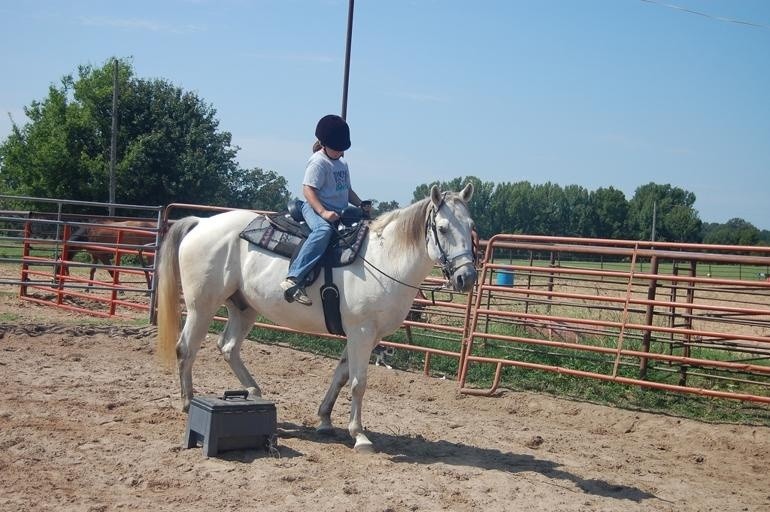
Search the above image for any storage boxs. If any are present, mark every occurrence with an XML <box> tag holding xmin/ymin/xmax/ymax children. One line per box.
<box><xmin>182</xmin><ymin>390</ymin><xmax>277</xmax><ymax>455</ymax></box>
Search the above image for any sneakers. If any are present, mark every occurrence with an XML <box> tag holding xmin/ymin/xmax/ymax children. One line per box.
<box><xmin>279</xmin><ymin>278</ymin><xmax>312</xmax><ymax>305</ymax></box>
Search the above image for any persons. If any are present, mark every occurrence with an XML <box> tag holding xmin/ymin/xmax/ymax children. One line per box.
<box><xmin>280</xmin><ymin>115</ymin><xmax>361</xmax><ymax>306</ymax></box>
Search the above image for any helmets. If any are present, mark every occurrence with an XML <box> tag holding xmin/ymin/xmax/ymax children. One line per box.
<box><xmin>315</xmin><ymin>115</ymin><xmax>350</xmax><ymax>151</ymax></box>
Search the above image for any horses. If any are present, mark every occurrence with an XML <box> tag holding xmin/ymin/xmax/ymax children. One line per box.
<box><xmin>156</xmin><ymin>182</ymin><xmax>478</xmax><ymax>455</ymax></box>
<box><xmin>50</xmin><ymin>217</ymin><xmax>170</xmax><ymax>296</ymax></box>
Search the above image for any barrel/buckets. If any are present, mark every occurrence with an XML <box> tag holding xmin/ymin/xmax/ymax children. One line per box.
<box><xmin>498</xmin><ymin>269</ymin><xmax>514</xmax><ymax>287</ymax></box>
